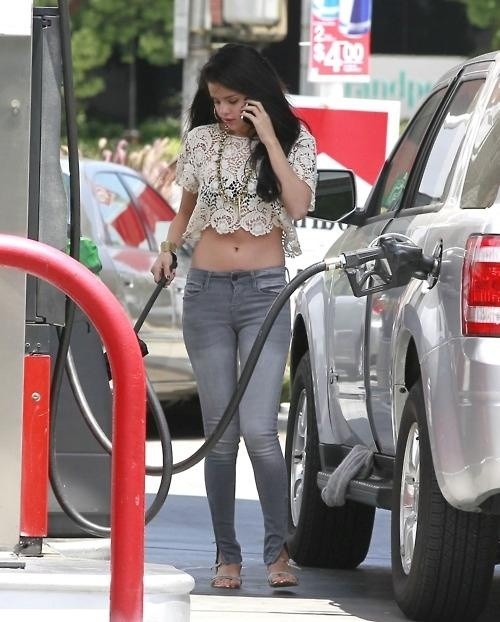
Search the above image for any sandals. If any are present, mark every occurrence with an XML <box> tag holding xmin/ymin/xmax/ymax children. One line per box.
<box><xmin>210</xmin><ymin>563</ymin><xmax>242</xmax><ymax>590</ymax></box>
<box><xmin>266</xmin><ymin>557</ymin><xmax>298</xmax><ymax>588</ymax></box>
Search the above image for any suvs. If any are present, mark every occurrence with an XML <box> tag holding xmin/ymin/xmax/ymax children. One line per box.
<box><xmin>279</xmin><ymin>50</ymin><xmax>500</xmax><ymax>622</ymax></box>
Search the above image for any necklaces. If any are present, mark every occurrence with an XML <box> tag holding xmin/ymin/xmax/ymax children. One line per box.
<box><xmin>217</xmin><ymin>123</ymin><xmax>255</xmax><ymax>201</ymax></box>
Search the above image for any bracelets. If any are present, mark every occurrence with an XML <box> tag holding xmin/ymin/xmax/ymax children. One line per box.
<box><xmin>160</xmin><ymin>242</ymin><xmax>178</xmax><ymax>255</ymax></box>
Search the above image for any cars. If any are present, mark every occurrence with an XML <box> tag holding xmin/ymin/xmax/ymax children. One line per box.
<box><xmin>59</xmin><ymin>156</ymin><xmax>197</xmax><ymax>406</ymax></box>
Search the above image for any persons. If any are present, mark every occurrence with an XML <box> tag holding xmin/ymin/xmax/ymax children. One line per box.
<box><xmin>151</xmin><ymin>42</ymin><xmax>318</xmax><ymax>589</ymax></box>
